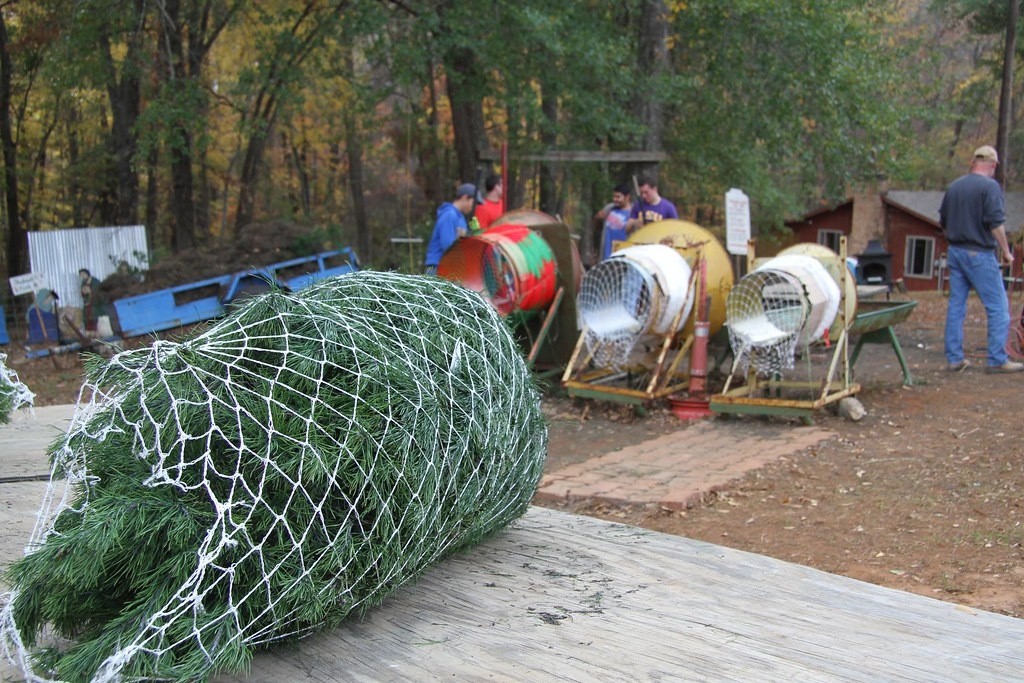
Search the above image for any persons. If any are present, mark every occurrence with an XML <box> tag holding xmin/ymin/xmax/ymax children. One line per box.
<box><xmin>465</xmin><ymin>172</ymin><xmax>502</xmax><ymax>234</ymax></box>
<box><xmin>424</xmin><ymin>182</ymin><xmax>484</xmax><ymax>277</ymax></box>
<box><xmin>593</xmin><ymin>183</ymin><xmax>643</xmax><ymax>263</ymax></box>
<box><xmin>937</xmin><ymin>143</ymin><xmax>1023</xmax><ymax>374</ymax></box>
<box><xmin>624</xmin><ymin>174</ymin><xmax>677</xmax><ymax>236</ymax></box>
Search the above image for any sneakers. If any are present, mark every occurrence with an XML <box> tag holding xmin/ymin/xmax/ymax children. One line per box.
<box><xmin>987</xmin><ymin>360</ymin><xmax>1023</xmax><ymax>373</ymax></box>
<box><xmin>949</xmin><ymin>359</ymin><xmax>969</xmax><ymax>372</ymax></box>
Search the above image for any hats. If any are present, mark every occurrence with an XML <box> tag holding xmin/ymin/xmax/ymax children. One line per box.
<box><xmin>456</xmin><ymin>183</ymin><xmax>483</xmax><ymax>204</ymax></box>
<box><xmin>974</xmin><ymin>145</ymin><xmax>1000</xmax><ymax>166</ymax></box>
